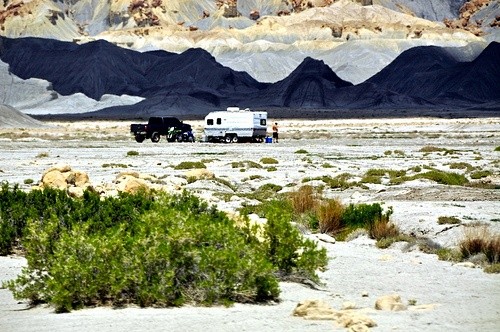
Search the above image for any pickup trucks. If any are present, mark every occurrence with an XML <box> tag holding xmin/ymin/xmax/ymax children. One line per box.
<box><xmin>130</xmin><ymin>116</ymin><xmax>191</xmax><ymax>142</ymax></box>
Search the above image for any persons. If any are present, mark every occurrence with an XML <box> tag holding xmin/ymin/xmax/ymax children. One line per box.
<box><xmin>272</xmin><ymin>122</ymin><xmax>279</xmax><ymax>143</ymax></box>
